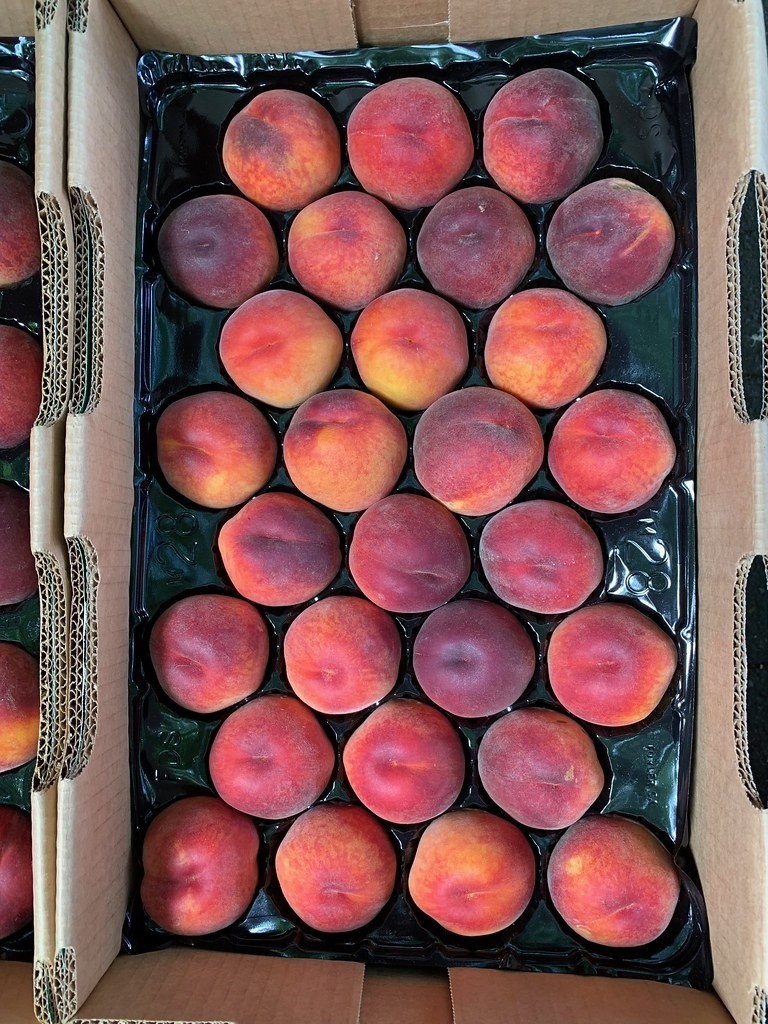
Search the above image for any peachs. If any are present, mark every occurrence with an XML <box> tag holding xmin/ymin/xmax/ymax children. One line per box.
<box><xmin>140</xmin><ymin>66</ymin><xmax>679</xmax><ymax>948</ymax></box>
<box><xmin>0</xmin><ymin>158</ymin><xmax>36</xmax><ymax>940</ymax></box>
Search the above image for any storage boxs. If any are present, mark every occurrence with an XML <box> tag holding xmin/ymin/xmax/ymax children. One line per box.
<box><xmin>0</xmin><ymin>0</ymin><xmax>768</xmax><ymax>1024</ymax></box>
